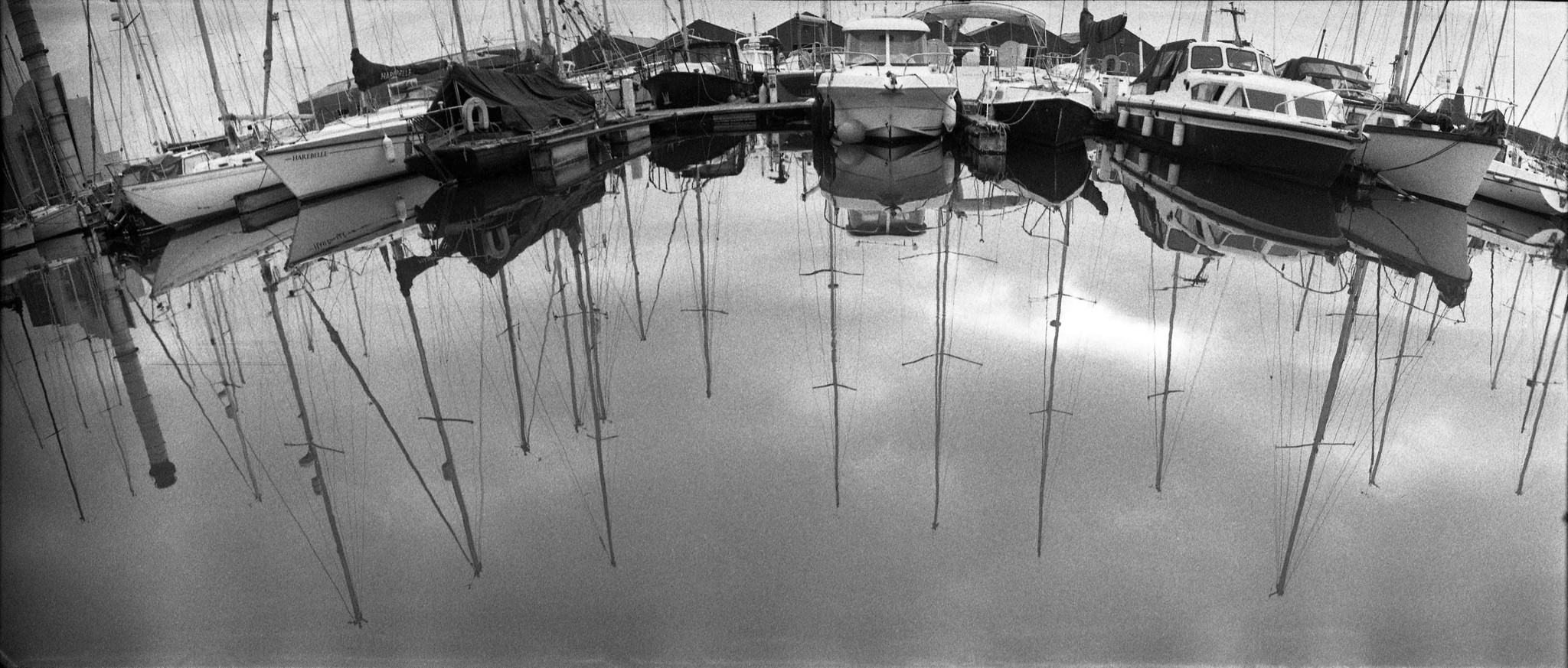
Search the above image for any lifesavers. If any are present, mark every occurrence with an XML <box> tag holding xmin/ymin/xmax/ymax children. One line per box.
<box><xmin>462</xmin><ymin>96</ymin><xmax>488</xmax><ymax>132</ymax></box>
<box><xmin>1101</xmin><ymin>55</ymin><xmax>1121</xmax><ymax>74</ymax></box>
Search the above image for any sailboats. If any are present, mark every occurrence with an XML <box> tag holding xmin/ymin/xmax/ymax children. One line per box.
<box><xmin>2</xmin><ymin>2</ymin><xmax>1568</xmax><ymax>250</ymax></box>
<box><xmin>2</xmin><ymin>139</ymin><xmax>1564</xmax><ymax>630</ymax></box>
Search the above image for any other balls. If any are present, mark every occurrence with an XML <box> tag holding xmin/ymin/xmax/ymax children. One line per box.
<box><xmin>837</xmin><ymin>118</ymin><xmax>864</xmax><ymax>142</ymax></box>
